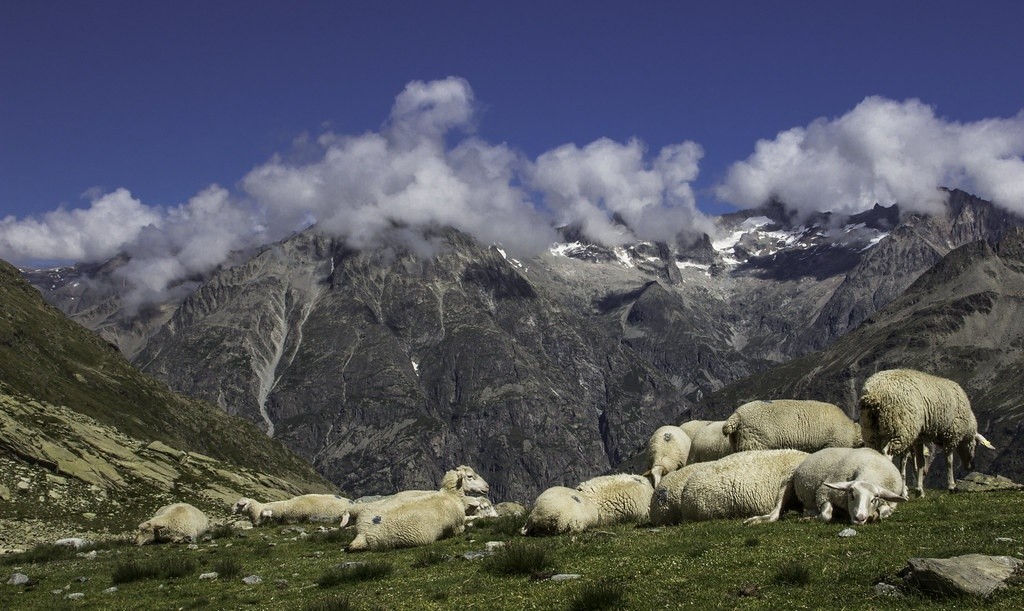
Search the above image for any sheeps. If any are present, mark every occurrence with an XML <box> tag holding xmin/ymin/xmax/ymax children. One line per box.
<box><xmin>134</xmin><ymin>502</ymin><xmax>209</xmax><ymax>546</ymax></box>
<box><xmin>230</xmin><ymin>368</ymin><xmax>997</xmax><ymax>552</ymax></box>
